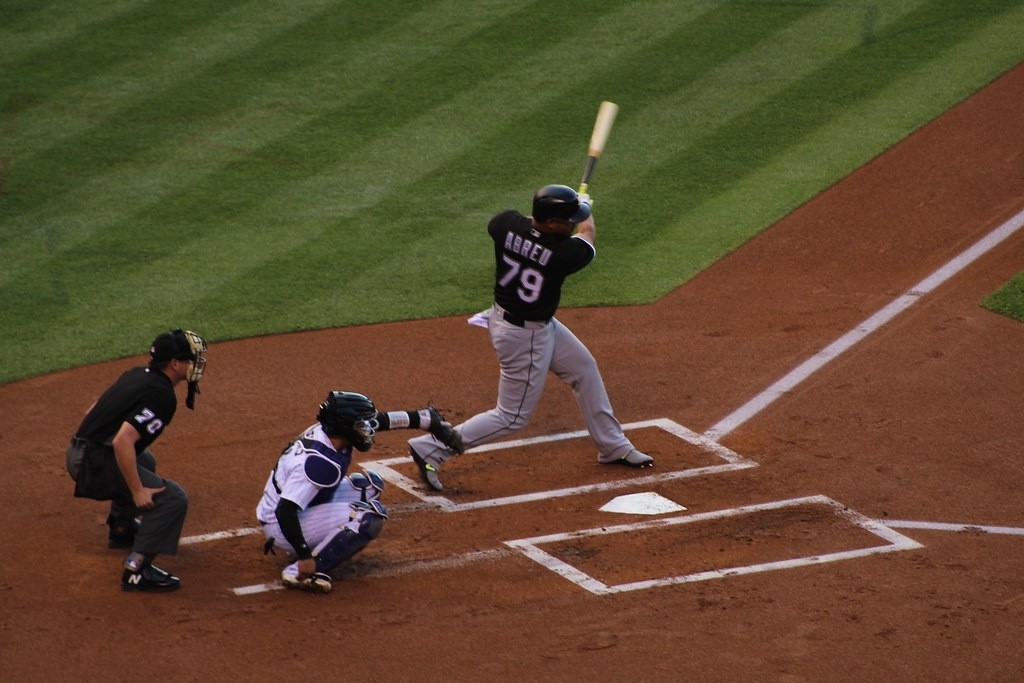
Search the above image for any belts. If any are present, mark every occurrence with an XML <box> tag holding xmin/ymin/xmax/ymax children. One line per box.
<box><xmin>260</xmin><ymin>521</ymin><xmax>267</xmax><ymax>526</ymax></box>
<box><xmin>72</xmin><ymin>437</ymin><xmax>87</xmax><ymax>449</ymax></box>
<box><xmin>493</xmin><ymin>301</ymin><xmax>553</xmax><ymax>327</ymax></box>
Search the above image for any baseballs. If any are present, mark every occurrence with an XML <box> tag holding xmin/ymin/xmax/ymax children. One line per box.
<box><xmin>579</xmin><ymin>98</ymin><xmax>622</xmax><ymax>193</ymax></box>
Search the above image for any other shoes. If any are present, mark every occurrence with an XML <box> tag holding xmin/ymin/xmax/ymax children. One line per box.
<box><xmin>282</xmin><ymin>564</ymin><xmax>333</xmax><ymax>594</ymax></box>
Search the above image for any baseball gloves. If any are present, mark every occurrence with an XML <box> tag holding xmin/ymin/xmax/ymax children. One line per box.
<box><xmin>430</xmin><ymin>409</ymin><xmax>466</xmax><ymax>455</ymax></box>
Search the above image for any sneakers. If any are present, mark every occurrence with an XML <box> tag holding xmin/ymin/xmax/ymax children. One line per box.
<box><xmin>121</xmin><ymin>562</ymin><xmax>181</xmax><ymax>592</ymax></box>
<box><xmin>619</xmin><ymin>447</ymin><xmax>655</xmax><ymax>468</ymax></box>
<box><xmin>109</xmin><ymin>518</ymin><xmax>143</xmax><ymax>548</ymax></box>
<box><xmin>407</xmin><ymin>443</ymin><xmax>443</xmax><ymax>492</ymax></box>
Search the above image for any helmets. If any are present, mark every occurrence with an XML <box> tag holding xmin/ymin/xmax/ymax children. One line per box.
<box><xmin>532</xmin><ymin>184</ymin><xmax>591</xmax><ymax>224</ymax></box>
<box><xmin>315</xmin><ymin>388</ymin><xmax>380</xmax><ymax>452</ymax></box>
<box><xmin>149</xmin><ymin>328</ymin><xmax>195</xmax><ymax>370</ymax></box>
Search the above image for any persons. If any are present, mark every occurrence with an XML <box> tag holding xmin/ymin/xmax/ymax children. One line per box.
<box><xmin>256</xmin><ymin>390</ymin><xmax>465</xmax><ymax>592</ymax></box>
<box><xmin>407</xmin><ymin>184</ymin><xmax>654</xmax><ymax>489</ymax></box>
<box><xmin>66</xmin><ymin>329</ymin><xmax>207</xmax><ymax>591</ymax></box>
